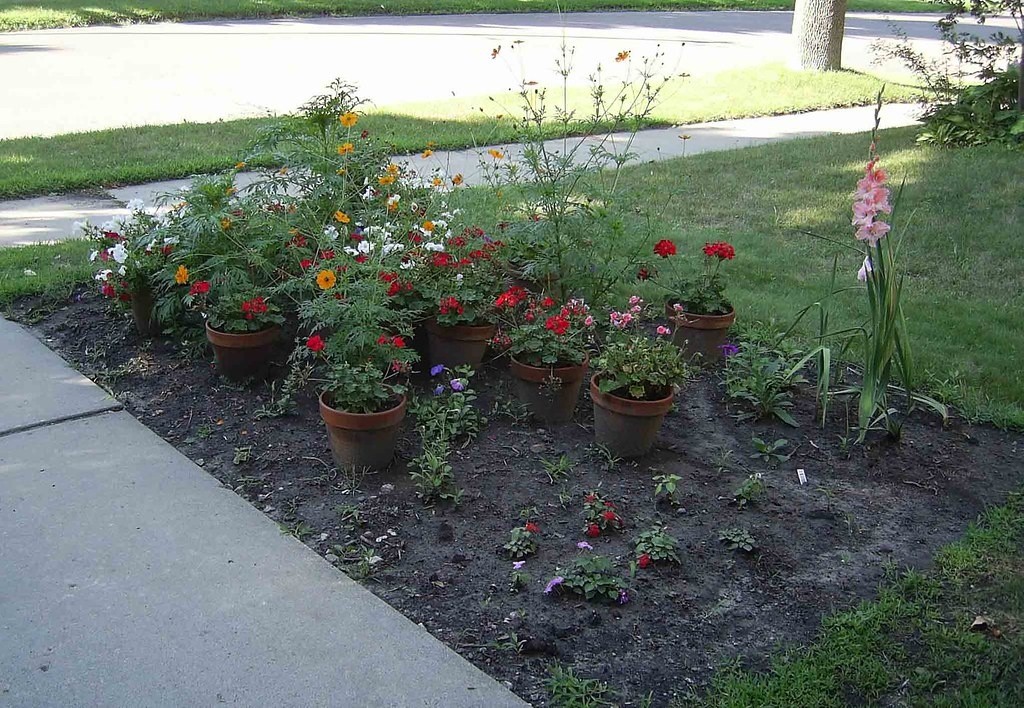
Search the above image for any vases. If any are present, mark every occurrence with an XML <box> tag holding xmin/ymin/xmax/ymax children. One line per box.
<box><xmin>589</xmin><ymin>373</ymin><xmax>674</xmax><ymax>458</ymax></box>
<box><xmin>130</xmin><ymin>291</ymin><xmax>180</xmax><ymax>353</ymax></box>
<box><xmin>664</xmin><ymin>295</ymin><xmax>734</xmax><ymax>365</ymax></box>
<box><xmin>508</xmin><ymin>348</ymin><xmax>586</xmax><ymax>422</ymax></box>
<box><xmin>205</xmin><ymin>315</ymin><xmax>276</xmax><ymax>375</ymax></box>
<box><xmin>503</xmin><ymin>260</ymin><xmax>558</xmax><ymax>292</ymax></box>
<box><xmin>318</xmin><ymin>381</ymin><xmax>407</xmax><ymax>473</ymax></box>
<box><xmin>419</xmin><ymin>315</ymin><xmax>493</xmax><ymax>375</ymax></box>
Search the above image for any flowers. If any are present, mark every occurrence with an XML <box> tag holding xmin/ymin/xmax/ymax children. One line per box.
<box><xmin>177</xmin><ymin>250</ymin><xmax>273</xmax><ymax>314</ymax></box>
<box><xmin>496</xmin><ymin>201</ymin><xmax>548</xmax><ymax>257</ymax></box>
<box><xmin>411</xmin><ymin>205</ymin><xmax>502</xmax><ymax>314</ymax></box>
<box><xmin>582</xmin><ymin>295</ymin><xmax>705</xmax><ymax>399</ymax></box>
<box><xmin>489</xmin><ymin>286</ymin><xmax>581</xmax><ymax>347</ymax></box>
<box><xmin>301</xmin><ymin>268</ymin><xmax>418</xmax><ymax>381</ymax></box>
<box><xmin>637</xmin><ymin>239</ymin><xmax>736</xmax><ymax>294</ymax></box>
<box><xmin>95</xmin><ymin>201</ymin><xmax>161</xmax><ymax>300</ymax></box>
